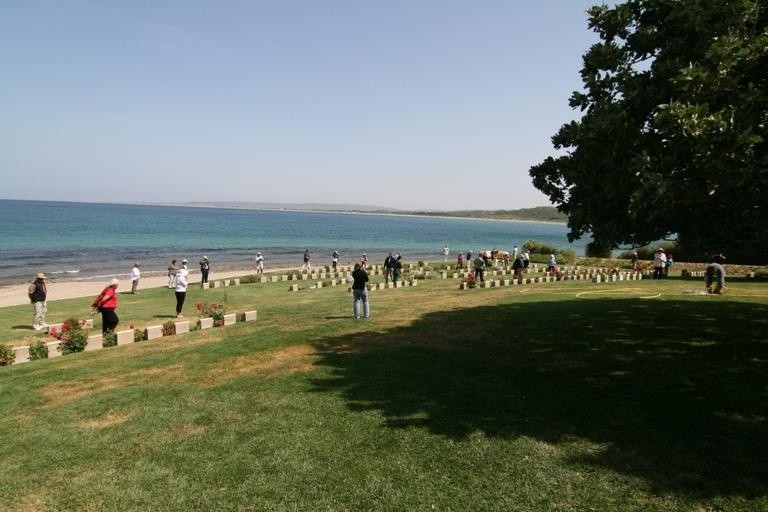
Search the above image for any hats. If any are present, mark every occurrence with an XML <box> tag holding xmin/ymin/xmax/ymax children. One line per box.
<box><xmin>177</xmin><ymin>269</ymin><xmax>187</xmax><ymax>275</ymax></box>
<box><xmin>107</xmin><ymin>278</ymin><xmax>119</xmax><ymax>285</ymax></box>
<box><xmin>34</xmin><ymin>274</ymin><xmax>47</xmax><ymax>278</ymax></box>
<box><xmin>656</xmin><ymin>248</ymin><xmax>664</xmax><ymax>251</ymax></box>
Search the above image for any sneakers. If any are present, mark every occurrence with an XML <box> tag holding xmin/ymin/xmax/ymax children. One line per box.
<box><xmin>33</xmin><ymin>324</ymin><xmax>47</xmax><ymax>330</ymax></box>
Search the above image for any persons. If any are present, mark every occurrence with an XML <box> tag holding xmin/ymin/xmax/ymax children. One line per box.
<box><xmin>653</xmin><ymin>248</ymin><xmax>667</xmax><ymax>279</ymax></box>
<box><xmin>131</xmin><ymin>262</ymin><xmax>142</xmax><ymax>294</ymax></box>
<box><xmin>168</xmin><ymin>258</ymin><xmax>190</xmax><ymax>319</ymax></box>
<box><xmin>632</xmin><ymin>252</ymin><xmax>638</xmax><ymax>271</ymax></box>
<box><xmin>712</xmin><ymin>250</ymin><xmax>727</xmax><ymax>265</ymax></box>
<box><xmin>255</xmin><ymin>252</ymin><xmax>264</xmax><ymax>277</ymax></box>
<box><xmin>28</xmin><ymin>273</ymin><xmax>49</xmax><ymax>330</ymax></box>
<box><xmin>706</xmin><ymin>262</ymin><xmax>726</xmax><ymax>294</ymax></box>
<box><xmin>455</xmin><ymin>245</ymin><xmax>529</xmax><ymax>283</ymax></box>
<box><xmin>302</xmin><ymin>248</ymin><xmax>312</xmax><ymax>271</ymax></box>
<box><xmin>548</xmin><ymin>254</ymin><xmax>556</xmax><ymax>271</ymax></box>
<box><xmin>352</xmin><ymin>252</ymin><xmax>370</xmax><ymax>319</ymax></box>
<box><xmin>332</xmin><ymin>250</ymin><xmax>339</xmax><ymax>268</ymax></box>
<box><xmin>382</xmin><ymin>251</ymin><xmax>404</xmax><ymax>288</ymax></box>
<box><xmin>199</xmin><ymin>256</ymin><xmax>210</xmax><ymax>283</ymax></box>
<box><xmin>441</xmin><ymin>244</ymin><xmax>449</xmax><ymax>263</ymax></box>
<box><xmin>91</xmin><ymin>278</ymin><xmax>119</xmax><ymax>338</ymax></box>
<box><xmin>665</xmin><ymin>253</ymin><xmax>673</xmax><ymax>278</ymax></box>
